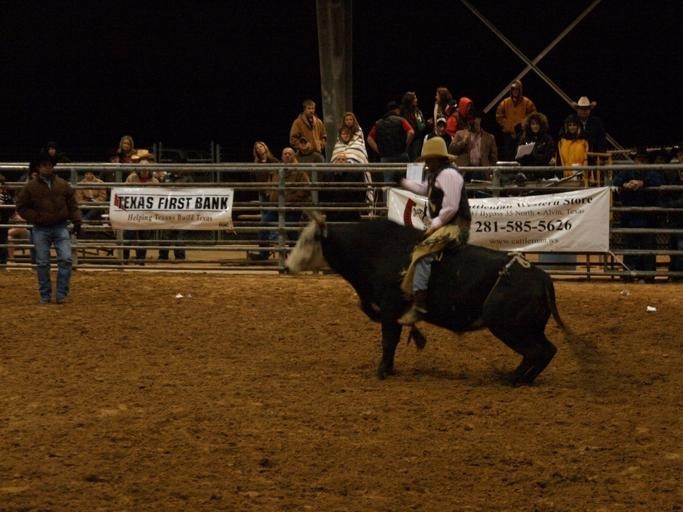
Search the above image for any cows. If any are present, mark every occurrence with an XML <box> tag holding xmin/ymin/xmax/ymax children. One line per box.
<box><xmin>282</xmin><ymin>206</ymin><xmax>565</xmax><ymax>388</ymax></box>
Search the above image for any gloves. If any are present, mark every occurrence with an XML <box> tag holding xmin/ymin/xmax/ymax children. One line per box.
<box><xmin>72</xmin><ymin>224</ymin><xmax>80</xmax><ymax>237</ymax></box>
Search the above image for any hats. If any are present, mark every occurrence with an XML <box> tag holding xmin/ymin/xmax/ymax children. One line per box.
<box><xmin>30</xmin><ymin>153</ymin><xmax>57</xmax><ymax>169</ymax></box>
<box><xmin>630</xmin><ymin>148</ymin><xmax>683</xmax><ymax>158</ymax></box>
<box><xmin>416</xmin><ymin>137</ymin><xmax>453</xmax><ymax>159</ymax></box>
<box><xmin>132</xmin><ymin>149</ymin><xmax>151</xmax><ymax>159</ymax></box>
<box><xmin>571</xmin><ymin>96</ymin><xmax>596</xmax><ymax>110</ymax></box>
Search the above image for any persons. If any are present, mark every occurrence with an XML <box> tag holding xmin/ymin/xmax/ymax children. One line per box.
<box><xmin>391</xmin><ymin>136</ymin><xmax>472</xmax><ymax>324</ymax></box>
<box><xmin>660</xmin><ymin>146</ymin><xmax>682</xmax><ymax>283</ymax></box>
<box><xmin>612</xmin><ymin>148</ymin><xmax>658</xmax><ymax>284</ymax></box>
<box><xmin>1</xmin><ymin>136</ymin><xmax>193</xmax><ymax>302</ymax></box>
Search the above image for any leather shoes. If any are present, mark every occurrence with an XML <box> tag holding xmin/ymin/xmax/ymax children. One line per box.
<box><xmin>396</xmin><ymin>305</ymin><xmax>427</xmax><ymax>324</ymax></box>
<box><xmin>40</xmin><ymin>300</ymin><xmax>48</xmax><ymax>303</ymax></box>
<box><xmin>56</xmin><ymin>299</ymin><xmax>64</xmax><ymax>303</ymax></box>
